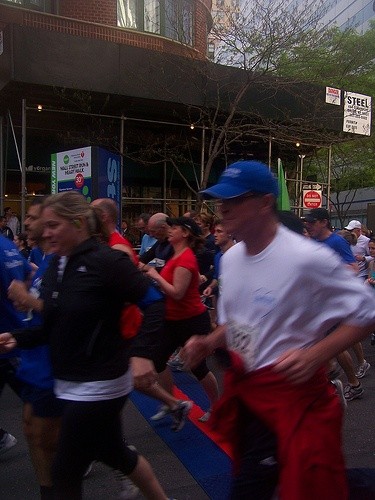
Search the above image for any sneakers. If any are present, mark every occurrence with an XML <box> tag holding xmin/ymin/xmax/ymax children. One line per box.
<box><xmin>161</xmin><ymin>399</ymin><xmax>193</xmax><ymax>434</ymax></box>
<box><xmin>344</xmin><ymin>381</ymin><xmax>365</xmax><ymax>400</ymax></box>
<box><xmin>328</xmin><ymin>363</ymin><xmax>344</xmax><ymax>380</ymax></box>
<box><xmin>355</xmin><ymin>359</ymin><xmax>370</xmax><ymax>379</ymax></box>
<box><xmin>332</xmin><ymin>379</ymin><xmax>347</xmax><ymax>408</ymax></box>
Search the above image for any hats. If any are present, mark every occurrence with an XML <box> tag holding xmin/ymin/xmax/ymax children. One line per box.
<box><xmin>301</xmin><ymin>207</ymin><xmax>330</xmax><ymax>222</ymax></box>
<box><xmin>4</xmin><ymin>207</ymin><xmax>11</xmax><ymax>216</ymax></box>
<box><xmin>166</xmin><ymin>216</ymin><xmax>200</xmax><ymax>238</ymax></box>
<box><xmin>199</xmin><ymin>160</ymin><xmax>280</xmax><ymax>201</ymax></box>
<box><xmin>344</xmin><ymin>219</ymin><xmax>362</xmax><ymax>230</ymax></box>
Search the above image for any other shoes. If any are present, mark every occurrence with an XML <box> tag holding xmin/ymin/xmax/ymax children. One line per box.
<box><xmin>370</xmin><ymin>332</ymin><xmax>375</xmax><ymax>345</ymax></box>
<box><xmin>118</xmin><ymin>445</ymin><xmax>143</xmax><ymax>500</ymax></box>
<box><xmin>151</xmin><ymin>410</ymin><xmax>166</xmax><ymax>420</ymax></box>
<box><xmin>198</xmin><ymin>410</ymin><xmax>211</xmax><ymax>422</ymax></box>
<box><xmin>259</xmin><ymin>455</ymin><xmax>279</xmax><ymax>466</ymax></box>
<box><xmin>165</xmin><ymin>351</ymin><xmax>185</xmax><ymax>371</ymax></box>
<box><xmin>0</xmin><ymin>433</ymin><xmax>18</xmax><ymax>453</ymax></box>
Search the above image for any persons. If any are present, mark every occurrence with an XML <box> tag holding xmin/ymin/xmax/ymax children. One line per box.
<box><xmin>0</xmin><ymin>191</ymin><xmax>171</xmax><ymax>500</ymax></box>
<box><xmin>139</xmin><ymin>208</ymin><xmax>375</xmax><ymax>422</ymax></box>
<box><xmin>180</xmin><ymin>161</ymin><xmax>375</xmax><ymax>500</ymax></box>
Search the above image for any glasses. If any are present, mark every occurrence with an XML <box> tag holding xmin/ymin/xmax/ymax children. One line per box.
<box><xmin>12</xmin><ymin>212</ymin><xmax>16</xmax><ymax>214</ymax></box>
<box><xmin>24</xmin><ymin>213</ymin><xmax>38</xmax><ymax>221</ymax></box>
<box><xmin>217</xmin><ymin>193</ymin><xmax>260</xmax><ymax>204</ymax></box>
